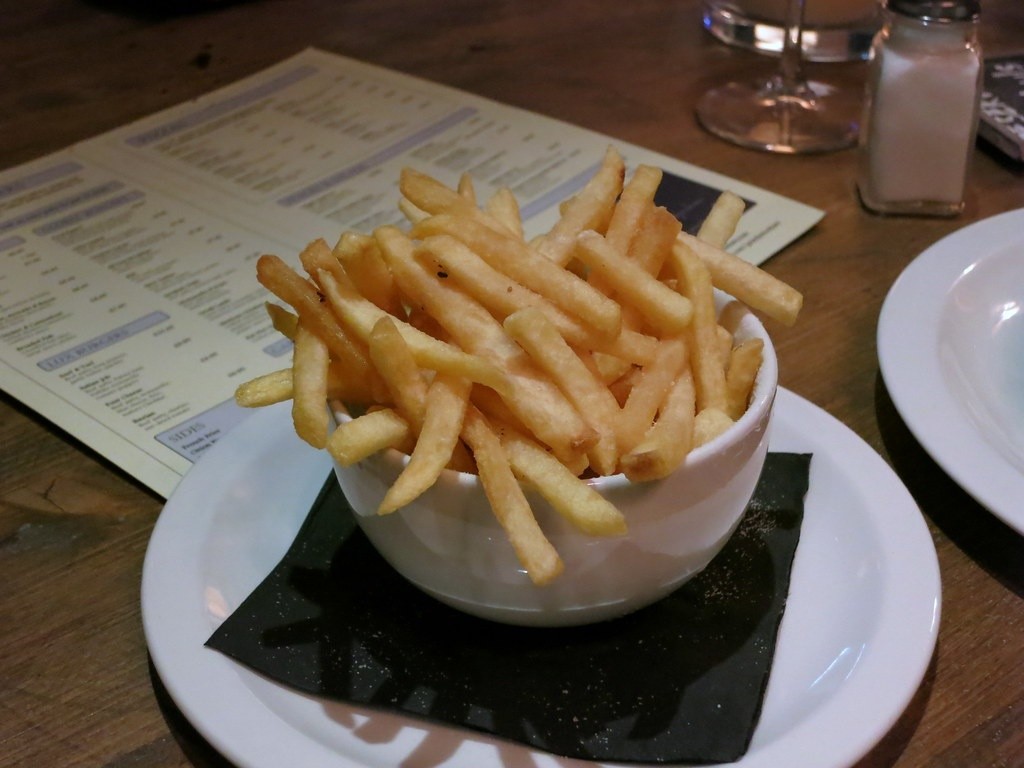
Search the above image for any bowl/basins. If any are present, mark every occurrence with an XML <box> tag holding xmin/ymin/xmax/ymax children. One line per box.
<box><xmin>306</xmin><ymin>279</ymin><xmax>778</xmax><ymax>621</ymax></box>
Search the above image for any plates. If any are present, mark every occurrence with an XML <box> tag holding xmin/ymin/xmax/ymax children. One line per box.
<box><xmin>878</xmin><ymin>208</ymin><xmax>1024</xmax><ymax>531</ymax></box>
<box><xmin>140</xmin><ymin>381</ymin><xmax>942</xmax><ymax>768</ymax></box>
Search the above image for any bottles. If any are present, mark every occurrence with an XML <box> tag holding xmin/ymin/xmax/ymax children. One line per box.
<box><xmin>862</xmin><ymin>0</ymin><xmax>983</xmax><ymax>213</ymax></box>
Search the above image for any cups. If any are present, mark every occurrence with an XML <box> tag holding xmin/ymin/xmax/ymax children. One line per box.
<box><xmin>693</xmin><ymin>0</ymin><xmax>879</xmax><ymax>155</ymax></box>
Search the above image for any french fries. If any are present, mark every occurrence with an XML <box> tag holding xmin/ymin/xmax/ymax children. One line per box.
<box><xmin>236</xmin><ymin>148</ymin><xmax>804</xmax><ymax>585</ymax></box>
